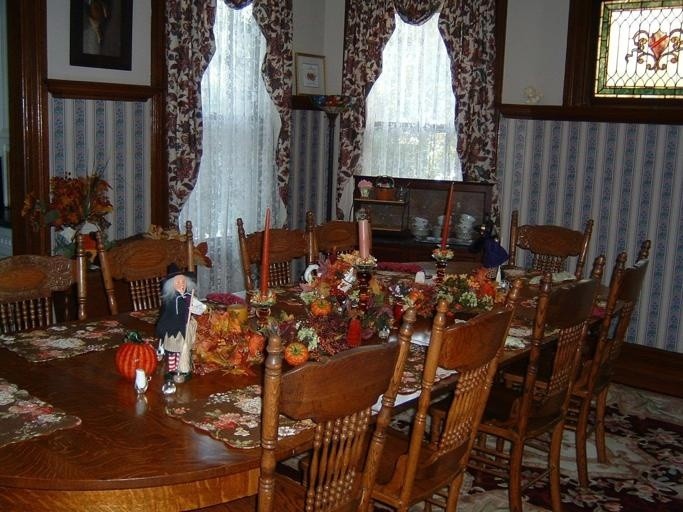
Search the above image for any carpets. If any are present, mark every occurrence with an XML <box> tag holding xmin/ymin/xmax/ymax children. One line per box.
<box><xmin>386</xmin><ymin>379</ymin><xmax>683</xmax><ymax>512</ymax></box>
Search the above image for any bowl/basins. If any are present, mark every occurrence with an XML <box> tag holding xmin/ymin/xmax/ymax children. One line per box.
<box><xmin>408</xmin><ymin>214</ymin><xmax>476</xmax><ymax>241</ymax></box>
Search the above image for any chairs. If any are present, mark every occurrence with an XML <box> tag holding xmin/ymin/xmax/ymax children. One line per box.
<box><xmin>426</xmin><ymin>251</ymin><xmax>607</xmax><ymax>511</ymax></box>
<box><xmin>508</xmin><ymin>209</ymin><xmax>594</xmax><ymax>279</ymax></box>
<box><xmin>298</xmin><ymin>279</ymin><xmax>524</xmax><ymax>511</ymax></box>
<box><xmin>1</xmin><ymin>233</ymin><xmax>87</xmax><ymax>335</ymax></box>
<box><xmin>305</xmin><ymin>212</ymin><xmax>374</xmax><ymax>277</ymax></box>
<box><xmin>195</xmin><ymin>306</ymin><xmax>417</xmax><ymax>511</ymax></box>
<box><xmin>97</xmin><ymin>220</ymin><xmax>194</xmax><ymax>317</ymax></box>
<box><xmin>492</xmin><ymin>239</ymin><xmax>651</xmax><ymax>491</ymax></box>
<box><xmin>237</xmin><ymin>210</ymin><xmax>314</xmax><ymax>293</ymax></box>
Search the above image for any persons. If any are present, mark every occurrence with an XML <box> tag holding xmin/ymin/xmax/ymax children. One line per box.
<box><xmin>154</xmin><ymin>263</ymin><xmax>197</xmax><ymax>380</ymax></box>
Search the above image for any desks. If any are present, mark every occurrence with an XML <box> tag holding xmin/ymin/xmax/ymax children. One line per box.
<box><xmin>0</xmin><ymin>260</ymin><xmax>610</xmax><ymax>512</ymax></box>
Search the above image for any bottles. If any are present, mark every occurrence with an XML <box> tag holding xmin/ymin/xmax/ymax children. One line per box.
<box><xmin>161</xmin><ymin>380</ymin><xmax>176</xmax><ymax>395</ymax></box>
<box><xmin>414</xmin><ymin>271</ymin><xmax>426</xmax><ymax>283</ymax></box>
<box><xmin>190</xmin><ymin>346</ymin><xmax>201</xmax><ymax>373</ymax></box>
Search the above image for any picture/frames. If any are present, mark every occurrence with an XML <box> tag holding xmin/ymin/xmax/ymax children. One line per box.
<box><xmin>293</xmin><ymin>50</ymin><xmax>327</xmax><ymax>98</ymax></box>
<box><xmin>43</xmin><ymin>1</ymin><xmax>162</xmax><ymax>95</ymax></box>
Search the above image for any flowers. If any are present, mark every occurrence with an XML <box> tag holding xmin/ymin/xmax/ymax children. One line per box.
<box><xmin>20</xmin><ymin>159</ymin><xmax>116</xmax><ymax>270</ymax></box>
<box><xmin>190</xmin><ymin>256</ymin><xmax>514</xmax><ymax>375</ymax></box>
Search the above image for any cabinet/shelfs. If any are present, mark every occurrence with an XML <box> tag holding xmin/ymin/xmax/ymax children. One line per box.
<box><xmin>353</xmin><ymin>173</ymin><xmax>495</xmax><ymax>262</ymax></box>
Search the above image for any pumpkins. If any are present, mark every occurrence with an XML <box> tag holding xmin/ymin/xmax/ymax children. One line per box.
<box><xmin>115</xmin><ymin>342</ymin><xmax>158</xmax><ymax>379</ymax></box>
<box><xmin>284</xmin><ymin>342</ymin><xmax>308</xmax><ymax>367</ymax></box>
<box><xmin>311</xmin><ymin>300</ymin><xmax>332</xmax><ymax>317</ymax></box>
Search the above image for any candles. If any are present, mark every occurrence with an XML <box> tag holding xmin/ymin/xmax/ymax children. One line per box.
<box><xmin>441</xmin><ymin>180</ymin><xmax>454</xmax><ymax>253</ymax></box>
<box><xmin>359</xmin><ymin>216</ymin><xmax>370</xmax><ymax>261</ymax></box>
<box><xmin>260</xmin><ymin>207</ymin><xmax>270</xmax><ymax>301</ymax></box>
